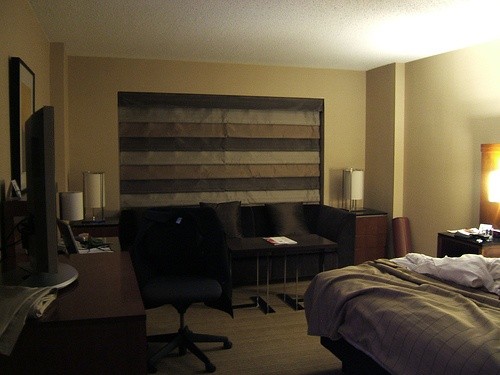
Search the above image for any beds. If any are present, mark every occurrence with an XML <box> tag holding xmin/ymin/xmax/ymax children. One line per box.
<box><xmin>303</xmin><ymin>255</ymin><xmax>500</xmax><ymax>375</ymax></box>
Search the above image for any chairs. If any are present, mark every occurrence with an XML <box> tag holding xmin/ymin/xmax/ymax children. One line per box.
<box><xmin>134</xmin><ymin>207</ymin><xmax>234</xmax><ymax>375</ymax></box>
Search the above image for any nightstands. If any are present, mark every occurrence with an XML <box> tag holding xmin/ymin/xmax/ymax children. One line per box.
<box><xmin>437</xmin><ymin>231</ymin><xmax>500</xmax><ymax>258</ymax></box>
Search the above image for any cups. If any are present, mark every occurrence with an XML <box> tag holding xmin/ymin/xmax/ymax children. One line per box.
<box><xmin>482</xmin><ymin>227</ymin><xmax>493</xmax><ymax>241</ymax></box>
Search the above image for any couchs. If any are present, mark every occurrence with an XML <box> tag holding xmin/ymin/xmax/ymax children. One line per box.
<box><xmin>121</xmin><ymin>204</ymin><xmax>349</xmax><ymax>287</ymax></box>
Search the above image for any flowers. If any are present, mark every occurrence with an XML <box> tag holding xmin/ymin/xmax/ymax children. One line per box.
<box><xmin>76</xmin><ymin>232</ymin><xmax>118</xmax><ymax>254</ymax></box>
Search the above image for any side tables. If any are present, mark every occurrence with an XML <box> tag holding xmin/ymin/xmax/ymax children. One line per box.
<box><xmin>70</xmin><ymin>216</ymin><xmax>120</xmax><ymax>237</ymax></box>
<box><xmin>343</xmin><ymin>208</ymin><xmax>388</xmax><ymax>266</ymax></box>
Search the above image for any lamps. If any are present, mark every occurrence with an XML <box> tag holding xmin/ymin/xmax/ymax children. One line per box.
<box><xmin>343</xmin><ymin>168</ymin><xmax>364</xmax><ymax>212</ymax></box>
<box><xmin>82</xmin><ymin>170</ymin><xmax>106</xmax><ymax>224</ymax></box>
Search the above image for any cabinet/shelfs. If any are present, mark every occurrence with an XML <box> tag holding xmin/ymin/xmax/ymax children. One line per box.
<box><xmin>0</xmin><ymin>251</ymin><xmax>148</xmax><ymax>375</ymax></box>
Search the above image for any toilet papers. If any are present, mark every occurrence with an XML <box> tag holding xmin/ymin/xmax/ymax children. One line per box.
<box><xmin>60</xmin><ymin>191</ymin><xmax>84</xmax><ymax>221</ymax></box>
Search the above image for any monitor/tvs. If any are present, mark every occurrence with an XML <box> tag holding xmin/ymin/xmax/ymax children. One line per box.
<box><xmin>17</xmin><ymin>104</ymin><xmax>79</xmax><ymax>288</ymax></box>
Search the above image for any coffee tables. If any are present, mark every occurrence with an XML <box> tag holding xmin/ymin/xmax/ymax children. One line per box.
<box><xmin>227</xmin><ymin>233</ymin><xmax>339</xmax><ymax>315</ymax></box>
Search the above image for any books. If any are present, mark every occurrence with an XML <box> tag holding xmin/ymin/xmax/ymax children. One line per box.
<box><xmin>263</xmin><ymin>236</ymin><xmax>297</xmax><ymax>246</ymax></box>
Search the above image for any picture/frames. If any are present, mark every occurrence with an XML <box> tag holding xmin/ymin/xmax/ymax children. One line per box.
<box><xmin>9</xmin><ymin>56</ymin><xmax>36</xmax><ymax>196</ymax></box>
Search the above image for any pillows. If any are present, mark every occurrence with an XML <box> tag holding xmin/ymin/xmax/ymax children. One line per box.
<box><xmin>200</xmin><ymin>201</ymin><xmax>242</xmax><ymax>239</ymax></box>
<box><xmin>265</xmin><ymin>202</ymin><xmax>309</xmax><ymax>236</ymax></box>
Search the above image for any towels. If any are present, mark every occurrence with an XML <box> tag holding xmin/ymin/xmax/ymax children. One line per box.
<box><xmin>389</xmin><ymin>252</ymin><xmax>500</xmax><ymax>296</ymax></box>
